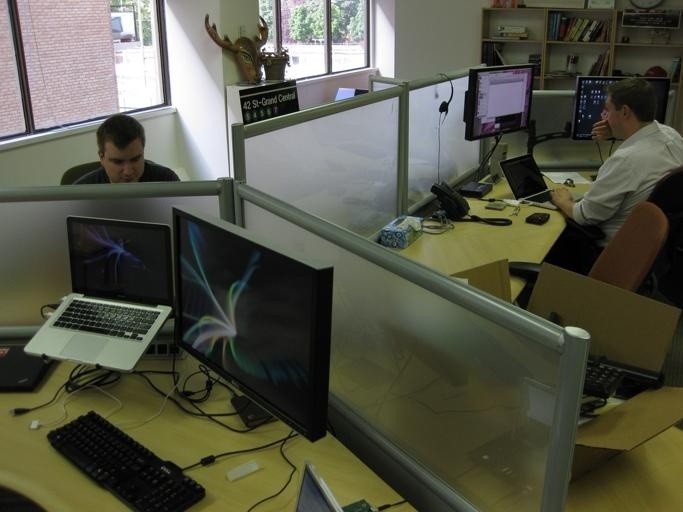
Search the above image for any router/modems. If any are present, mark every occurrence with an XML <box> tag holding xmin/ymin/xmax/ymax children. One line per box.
<box><xmin>0</xmin><ymin>346</ymin><xmax>53</xmax><ymax>392</ymax></box>
<box><xmin>459</xmin><ymin>181</ymin><xmax>493</xmax><ymax>200</ymax></box>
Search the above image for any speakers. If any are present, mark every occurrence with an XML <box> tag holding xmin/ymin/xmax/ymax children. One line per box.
<box><xmin>490</xmin><ymin>142</ymin><xmax>509</xmax><ymax>178</ymax></box>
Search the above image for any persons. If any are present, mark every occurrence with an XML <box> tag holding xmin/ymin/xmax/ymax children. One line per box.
<box><xmin>74</xmin><ymin>115</ymin><xmax>181</xmax><ymax>186</ymax></box>
<box><xmin>548</xmin><ymin>76</ymin><xmax>683</xmax><ymax>276</ymax></box>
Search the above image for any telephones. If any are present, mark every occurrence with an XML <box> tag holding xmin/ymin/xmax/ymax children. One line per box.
<box><xmin>430</xmin><ymin>181</ymin><xmax>512</xmax><ymax>226</ymax></box>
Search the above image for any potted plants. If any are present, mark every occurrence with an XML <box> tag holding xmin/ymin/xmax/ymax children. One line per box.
<box><xmin>260</xmin><ymin>46</ymin><xmax>289</xmax><ymax>81</ymax></box>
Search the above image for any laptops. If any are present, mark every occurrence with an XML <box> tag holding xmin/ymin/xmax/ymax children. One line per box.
<box><xmin>501</xmin><ymin>154</ymin><xmax>584</xmax><ymax>210</ymax></box>
<box><xmin>293</xmin><ymin>463</ymin><xmax>342</xmax><ymax>512</ymax></box>
<box><xmin>21</xmin><ymin>215</ymin><xmax>173</xmax><ymax>373</ymax></box>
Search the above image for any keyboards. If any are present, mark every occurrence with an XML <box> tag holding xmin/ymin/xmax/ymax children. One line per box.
<box><xmin>582</xmin><ymin>362</ymin><xmax>627</xmax><ymax>398</ymax></box>
<box><xmin>46</xmin><ymin>409</ymin><xmax>206</xmax><ymax>512</ymax></box>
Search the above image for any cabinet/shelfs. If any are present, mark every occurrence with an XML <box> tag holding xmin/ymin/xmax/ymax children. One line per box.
<box><xmin>477</xmin><ymin>9</ymin><xmax>683</xmax><ymax>92</ymax></box>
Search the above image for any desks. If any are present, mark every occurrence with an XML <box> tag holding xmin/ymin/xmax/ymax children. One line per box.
<box><xmin>568</xmin><ymin>423</ymin><xmax>683</xmax><ymax>512</ymax></box>
<box><xmin>376</xmin><ymin>167</ymin><xmax>605</xmax><ymax>308</ymax></box>
<box><xmin>0</xmin><ymin>332</ymin><xmax>422</xmax><ymax>511</ymax></box>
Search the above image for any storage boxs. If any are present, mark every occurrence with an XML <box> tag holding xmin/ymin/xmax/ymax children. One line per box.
<box><xmin>448</xmin><ymin>259</ymin><xmax>682</xmax><ymax>485</ymax></box>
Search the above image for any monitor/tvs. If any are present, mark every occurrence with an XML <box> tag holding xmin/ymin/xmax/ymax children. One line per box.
<box><xmin>367</xmin><ymin>75</ymin><xmax>404</xmax><ymax>93</ymax></box>
<box><xmin>572</xmin><ymin>75</ymin><xmax>670</xmax><ymax>141</ymax></box>
<box><xmin>168</xmin><ymin>202</ymin><xmax>335</xmax><ymax>444</ymax></box>
<box><xmin>464</xmin><ymin>63</ymin><xmax>534</xmax><ymax>141</ymax></box>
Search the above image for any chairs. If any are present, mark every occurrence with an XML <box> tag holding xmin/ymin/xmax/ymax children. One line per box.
<box><xmin>588</xmin><ymin>200</ymin><xmax>671</xmax><ymax>293</ymax></box>
<box><xmin>587</xmin><ymin>165</ymin><xmax>683</xmax><ymax>298</ymax></box>
<box><xmin>60</xmin><ymin>159</ymin><xmax>154</xmax><ymax>185</ymax></box>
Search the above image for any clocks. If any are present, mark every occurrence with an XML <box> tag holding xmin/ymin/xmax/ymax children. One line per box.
<box><xmin>629</xmin><ymin>0</ymin><xmax>664</xmax><ymax>8</ymax></box>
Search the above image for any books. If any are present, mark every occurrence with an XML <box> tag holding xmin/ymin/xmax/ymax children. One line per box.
<box><xmin>667</xmin><ymin>57</ymin><xmax>681</xmax><ymax>81</ymax></box>
<box><xmin>481</xmin><ymin>42</ymin><xmax>503</xmax><ymax>66</ymax></box>
<box><xmin>587</xmin><ymin>47</ymin><xmax>611</xmax><ymax>77</ymax></box>
<box><xmin>490</xmin><ymin>24</ymin><xmax>530</xmax><ymax>41</ymax></box>
<box><xmin>547</xmin><ymin>12</ymin><xmax>610</xmax><ymax>41</ymax></box>
